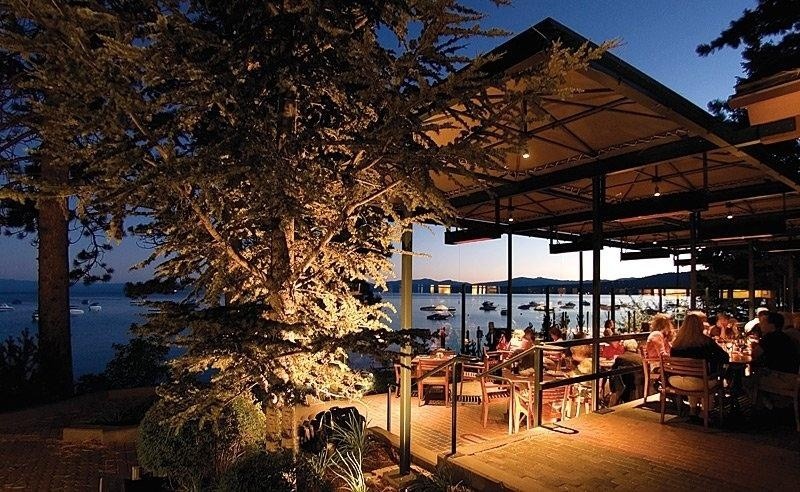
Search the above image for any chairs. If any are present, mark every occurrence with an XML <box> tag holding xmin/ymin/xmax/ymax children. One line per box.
<box><xmin>752</xmin><ymin>366</ymin><xmax>799</xmax><ymax>434</ymax></box>
<box><xmin>480</xmin><ymin>322</ymin><xmax>613</xmax><ymax>435</ymax></box>
<box><xmin>659</xmin><ymin>355</ymin><xmax>724</xmax><ymax>432</ymax></box>
<box><xmin>394</xmin><ymin>328</ymin><xmax>484</xmax><ymax>407</ymax></box>
<box><xmin>601</xmin><ymin>320</ymin><xmax>800</xmax><ymax>411</ymax></box>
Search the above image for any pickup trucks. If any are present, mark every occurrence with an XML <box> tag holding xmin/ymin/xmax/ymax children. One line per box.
<box><xmin>737</xmin><ymin>297</ymin><xmax>763</xmax><ymax>323</ymax></box>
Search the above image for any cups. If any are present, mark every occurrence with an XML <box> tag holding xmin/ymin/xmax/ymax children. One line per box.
<box><xmin>713</xmin><ymin>334</ymin><xmax>759</xmax><ymax>362</ymax></box>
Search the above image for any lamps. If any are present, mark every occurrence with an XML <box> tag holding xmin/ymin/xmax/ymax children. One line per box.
<box><xmin>726</xmin><ymin>206</ymin><xmax>734</xmax><ymax>221</ymax></box>
<box><xmin>653</xmin><ymin>181</ymin><xmax>661</xmax><ymax>197</ymax></box>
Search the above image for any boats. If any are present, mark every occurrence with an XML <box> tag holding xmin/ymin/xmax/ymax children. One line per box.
<box><xmin>517</xmin><ymin>298</ymin><xmax>629</xmax><ymax>312</ymax></box>
<box><xmin>500</xmin><ymin>309</ymin><xmax>507</xmax><ymax>316</ymax></box>
<box><xmin>0</xmin><ymin>304</ymin><xmax>16</xmax><ymax>313</ymax></box>
<box><xmin>80</xmin><ymin>298</ymin><xmax>90</xmax><ymax>306</ymax></box>
<box><xmin>187</xmin><ymin>298</ymin><xmax>197</xmax><ymax>305</ymax></box>
<box><xmin>70</xmin><ymin>305</ymin><xmax>84</xmax><ymax>315</ymax></box>
<box><xmin>427</xmin><ymin>311</ymin><xmax>453</xmax><ymax>322</ymax></box>
<box><xmin>32</xmin><ymin>310</ymin><xmax>39</xmax><ymax>322</ymax></box>
<box><xmin>148</xmin><ymin>307</ymin><xmax>167</xmax><ymax>314</ymax></box>
<box><xmin>419</xmin><ymin>304</ymin><xmax>457</xmax><ymax>312</ymax></box>
<box><xmin>87</xmin><ymin>302</ymin><xmax>102</xmax><ymax>312</ymax></box>
<box><xmin>479</xmin><ymin>301</ymin><xmax>497</xmax><ymax>311</ymax></box>
<box><xmin>129</xmin><ymin>297</ymin><xmax>146</xmax><ymax>306</ymax></box>
